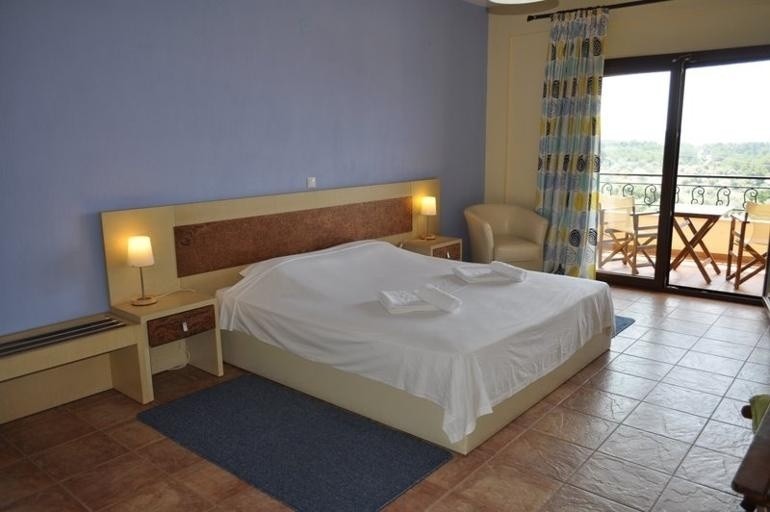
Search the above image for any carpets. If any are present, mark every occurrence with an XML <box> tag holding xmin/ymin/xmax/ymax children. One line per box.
<box><xmin>135</xmin><ymin>319</ymin><xmax>635</xmax><ymax>512</ymax></box>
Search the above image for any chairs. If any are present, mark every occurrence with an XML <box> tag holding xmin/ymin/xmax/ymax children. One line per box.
<box><xmin>599</xmin><ymin>197</ymin><xmax>655</xmax><ymax>272</ymax></box>
<box><xmin>727</xmin><ymin>204</ymin><xmax>770</xmax><ymax>289</ymax></box>
<box><xmin>462</xmin><ymin>201</ymin><xmax>549</xmax><ymax>271</ymax></box>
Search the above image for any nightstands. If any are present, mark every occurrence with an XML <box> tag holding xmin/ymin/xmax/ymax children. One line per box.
<box><xmin>409</xmin><ymin>234</ymin><xmax>463</xmax><ymax>262</ymax></box>
<box><xmin>113</xmin><ymin>291</ymin><xmax>225</xmax><ymax>404</ymax></box>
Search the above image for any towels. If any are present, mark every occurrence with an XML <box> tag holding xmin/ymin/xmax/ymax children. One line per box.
<box><xmin>450</xmin><ymin>261</ymin><xmax>526</xmax><ymax>284</ymax></box>
<box><xmin>374</xmin><ymin>280</ymin><xmax>461</xmax><ymax>316</ymax></box>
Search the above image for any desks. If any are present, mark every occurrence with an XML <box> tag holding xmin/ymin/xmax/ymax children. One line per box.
<box><xmin>0</xmin><ymin>314</ymin><xmax>148</xmax><ymax>430</ymax></box>
<box><xmin>657</xmin><ymin>202</ymin><xmax>728</xmax><ymax>283</ymax></box>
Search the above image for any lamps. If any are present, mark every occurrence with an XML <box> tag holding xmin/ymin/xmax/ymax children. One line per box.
<box><xmin>124</xmin><ymin>234</ymin><xmax>161</xmax><ymax>307</ymax></box>
<box><xmin>411</xmin><ymin>197</ymin><xmax>440</xmax><ymax>241</ymax></box>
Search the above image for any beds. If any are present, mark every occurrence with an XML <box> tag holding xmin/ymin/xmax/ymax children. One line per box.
<box><xmin>218</xmin><ymin>237</ymin><xmax>617</xmax><ymax>457</ymax></box>
